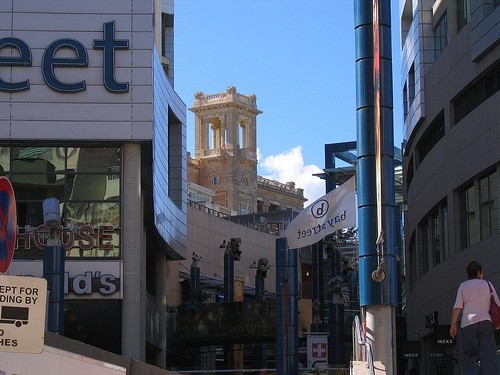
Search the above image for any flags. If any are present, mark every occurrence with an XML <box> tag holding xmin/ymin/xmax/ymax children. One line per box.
<box><xmin>284</xmin><ymin>174</ymin><xmax>357</xmax><ymax>251</ymax></box>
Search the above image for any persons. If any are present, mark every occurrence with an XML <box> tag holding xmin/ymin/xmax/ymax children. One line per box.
<box><xmin>450</xmin><ymin>260</ymin><xmax>500</xmax><ymax>375</ymax></box>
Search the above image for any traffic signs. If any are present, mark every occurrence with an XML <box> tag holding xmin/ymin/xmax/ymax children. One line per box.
<box><xmin>0</xmin><ymin>275</ymin><xmax>47</xmax><ymax>354</ymax></box>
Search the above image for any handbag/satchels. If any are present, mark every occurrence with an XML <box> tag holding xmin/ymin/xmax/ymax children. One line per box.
<box><xmin>489</xmin><ymin>295</ymin><xmax>500</xmax><ymax>330</ymax></box>
